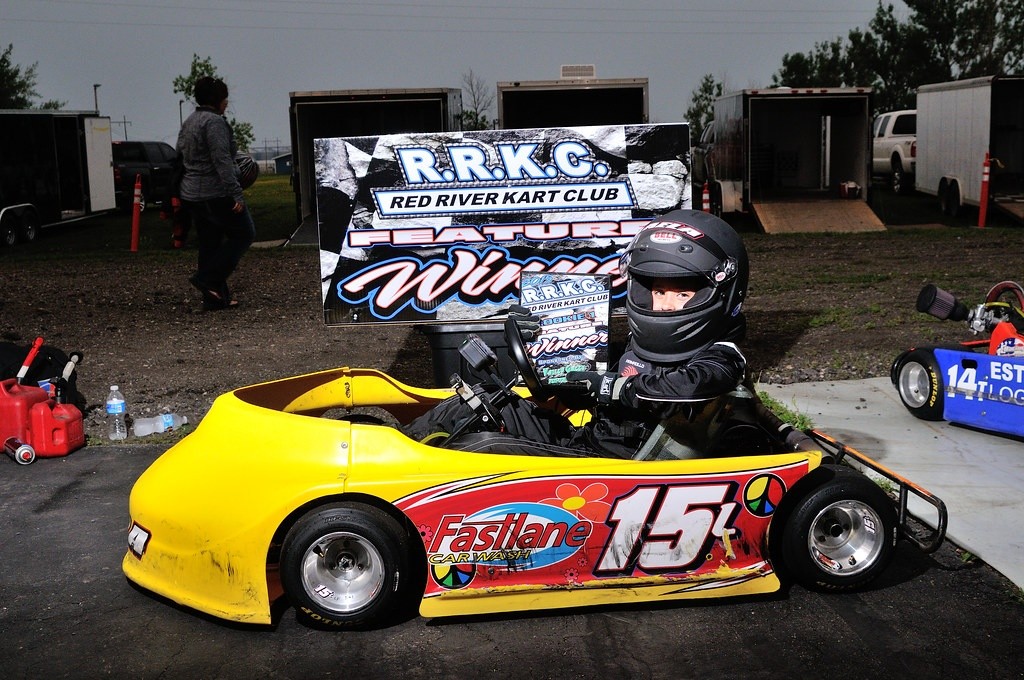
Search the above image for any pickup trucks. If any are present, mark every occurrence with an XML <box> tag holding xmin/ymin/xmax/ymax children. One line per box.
<box><xmin>111</xmin><ymin>140</ymin><xmax>176</xmax><ymax>213</ymax></box>
<box><xmin>872</xmin><ymin>110</ymin><xmax>917</xmax><ymax>192</ymax></box>
<box><xmin>691</xmin><ymin>121</ymin><xmax>715</xmax><ymax>188</ymax></box>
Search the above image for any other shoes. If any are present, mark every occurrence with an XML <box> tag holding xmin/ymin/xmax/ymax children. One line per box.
<box><xmin>188</xmin><ymin>273</ymin><xmax>239</xmax><ymax>310</ymax></box>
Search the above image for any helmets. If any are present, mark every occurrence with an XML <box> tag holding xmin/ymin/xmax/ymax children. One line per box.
<box><xmin>624</xmin><ymin>208</ymin><xmax>749</xmax><ymax>365</ymax></box>
<box><xmin>234</xmin><ymin>156</ymin><xmax>260</xmax><ymax>190</ymax></box>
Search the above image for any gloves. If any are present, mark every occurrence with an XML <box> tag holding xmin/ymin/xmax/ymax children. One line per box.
<box><xmin>507</xmin><ymin>302</ymin><xmax>545</xmax><ymax>341</ymax></box>
<box><xmin>567</xmin><ymin>368</ymin><xmax>621</xmax><ymax>405</ymax></box>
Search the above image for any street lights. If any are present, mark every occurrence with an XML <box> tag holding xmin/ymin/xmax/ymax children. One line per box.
<box><xmin>94</xmin><ymin>83</ymin><xmax>102</xmax><ymax>111</ymax></box>
<box><xmin>180</xmin><ymin>100</ymin><xmax>185</xmax><ymax>128</ymax></box>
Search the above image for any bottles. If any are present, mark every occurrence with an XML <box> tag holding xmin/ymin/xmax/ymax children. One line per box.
<box><xmin>106</xmin><ymin>385</ymin><xmax>127</xmax><ymax>441</ymax></box>
<box><xmin>134</xmin><ymin>414</ymin><xmax>188</xmax><ymax>436</ymax></box>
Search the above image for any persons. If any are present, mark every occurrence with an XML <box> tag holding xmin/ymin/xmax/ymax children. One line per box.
<box><xmin>175</xmin><ymin>76</ymin><xmax>255</xmax><ymax>308</ymax></box>
<box><xmin>405</xmin><ymin>210</ymin><xmax>753</xmax><ymax>457</ymax></box>
